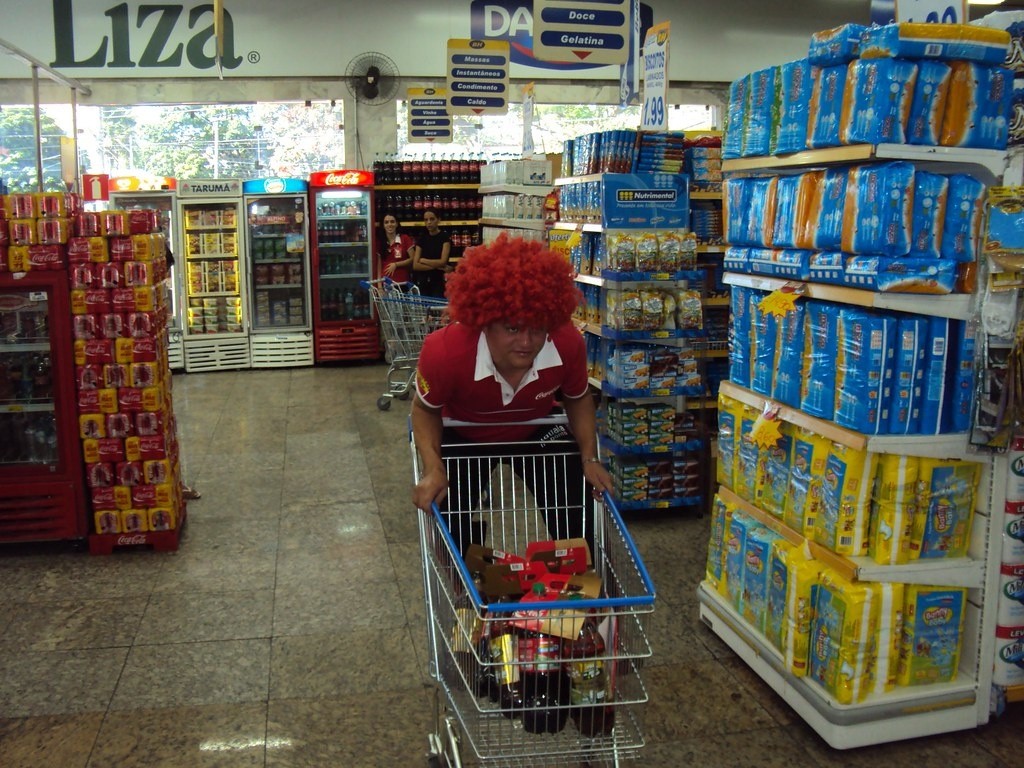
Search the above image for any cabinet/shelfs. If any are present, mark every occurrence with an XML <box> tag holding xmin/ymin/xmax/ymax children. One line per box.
<box><xmin>695</xmin><ymin>133</ymin><xmax>1023</xmax><ymax>750</ymax></box>
<box><xmin>552</xmin><ymin>172</ymin><xmax>728</xmax><ymax>519</ymax></box>
<box><xmin>475</xmin><ymin>186</ymin><xmax>553</xmax><ymax>232</ymax></box>
<box><xmin>375</xmin><ymin>184</ymin><xmax>479</xmax><ymax>264</ymax></box>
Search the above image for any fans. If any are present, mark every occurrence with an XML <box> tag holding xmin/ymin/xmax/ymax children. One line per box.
<box><xmin>345</xmin><ymin>52</ymin><xmax>401</xmax><ymax>106</ymax></box>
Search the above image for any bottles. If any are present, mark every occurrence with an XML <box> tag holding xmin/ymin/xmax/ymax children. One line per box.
<box><xmin>318</xmin><ymin>220</ymin><xmax>367</xmax><ymax>243</ymax></box>
<box><xmin>445</xmin><ymin>572</ymin><xmax>616</xmax><ymax>739</ymax></box>
<box><xmin>376</xmin><ymin>190</ymin><xmax>484</xmax><ymax>222</ymax></box>
<box><xmin>377</xmin><ymin>229</ymin><xmax>482</xmax><ymax>258</ymax></box>
<box><xmin>319</xmin><ymin>253</ymin><xmax>368</xmax><ymax>276</ymax></box>
<box><xmin>320</xmin><ymin>287</ymin><xmax>370</xmax><ymax>322</ymax></box>
<box><xmin>374</xmin><ymin>151</ymin><xmax>524</xmax><ymax>186</ymax></box>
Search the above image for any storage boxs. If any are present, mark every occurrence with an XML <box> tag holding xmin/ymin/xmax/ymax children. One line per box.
<box><xmin>478</xmin><ymin>160</ymin><xmax>554</xmax><ymax>244</ymax></box>
<box><xmin>585</xmin><ymin>332</ymin><xmax>726</xmax><ymax>500</ymax></box>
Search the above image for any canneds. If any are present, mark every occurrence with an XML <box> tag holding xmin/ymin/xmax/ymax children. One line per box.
<box><xmin>2</xmin><ymin>192</ymin><xmax>181</xmax><ymax>534</ymax></box>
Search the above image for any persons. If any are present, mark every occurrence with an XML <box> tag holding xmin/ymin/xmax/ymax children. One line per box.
<box><xmin>412</xmin><ymin>207</ymin><xmax>453</xmax><ymax>336</ymax></box>
<box><xmin>410</xmin><ymin>233</ymin><xmax>645</xmax><ymax>678</ymax></box>
<box><xmin>181</xmin><ymin>482</ymin><xmax>202</xmax><ymax>501</ymax></box>
<box><xmin>375</xmin><ymin>210</ymin><xmax>414</xmax><ymax>369</ymax></box>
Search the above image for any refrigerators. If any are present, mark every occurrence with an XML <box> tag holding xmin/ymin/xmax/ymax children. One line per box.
<box><xmin>107</xmin><ymin>175</ymin><xmax>184</xmax><ymax>372</ymax></box>
<box><xmin>309</xmin><ymin>169</ymin><xmax>378</xmax><ymax>367</ymax></box>
<box><xmin>242</xmin><ymin>178</ymin><xmax>314</xmax><ymax>369</ymax></box>
<box><xmin>176</xmin><ymin>178</ymin><xmax>250</xmax><ymax>373</ymax></box>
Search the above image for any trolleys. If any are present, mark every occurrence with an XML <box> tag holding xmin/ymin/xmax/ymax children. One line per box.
<box><xmin>406</xmin><ymin>412</ymin><xmax>656</xmax><ymax>768</ymax></box>
<box><xmin>360</xmin><ymin>277</ymin><xmax>461</xmax><ymax>411</ymax></box>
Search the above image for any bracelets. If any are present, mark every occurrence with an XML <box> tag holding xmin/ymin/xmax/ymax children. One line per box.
<box><xmin>582</xmin><ymin>456</ymin><xmax>600</xmax><ymax>467</ymax></box>
